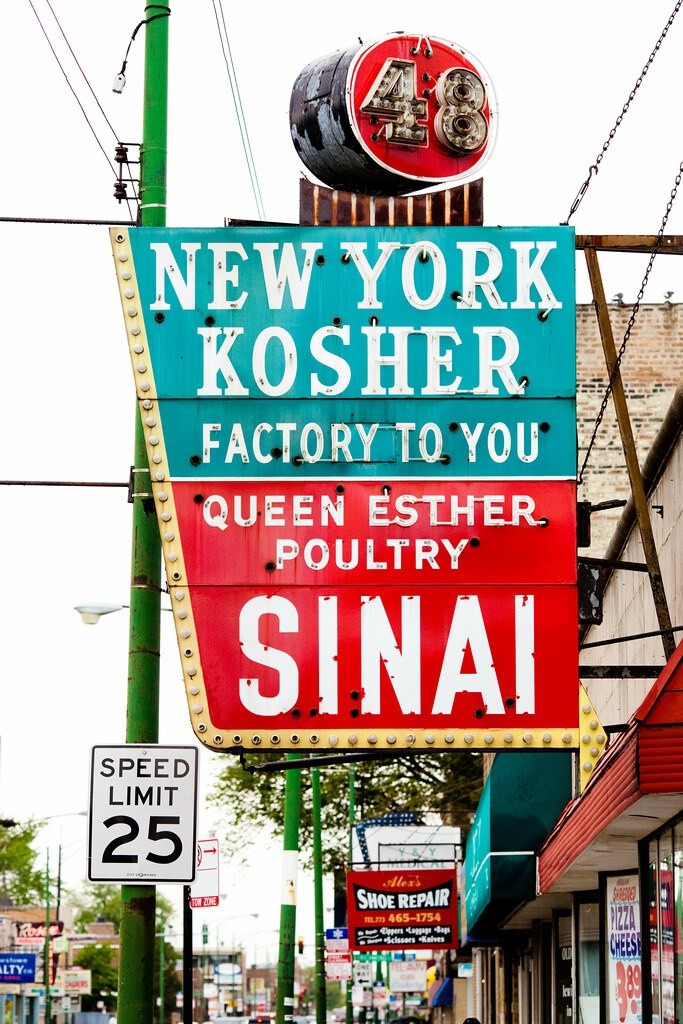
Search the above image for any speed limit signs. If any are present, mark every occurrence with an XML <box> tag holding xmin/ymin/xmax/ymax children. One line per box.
<box><xmin>87</xmin><ymin>741</ymin><xmax>194</xmax><ymax>884</ymax></box>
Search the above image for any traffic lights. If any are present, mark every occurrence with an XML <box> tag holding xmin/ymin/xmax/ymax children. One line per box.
<box><xmin>297</xmin><ymin>937</ymin><xmax>304</xmax><ymax>954</ymax></box>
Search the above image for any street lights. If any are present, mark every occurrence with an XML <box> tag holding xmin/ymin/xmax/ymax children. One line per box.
<box><xmin>204</xmin><ymin>911</ymin><xmax>258</xmax><ymax>1020</ymax></box>
